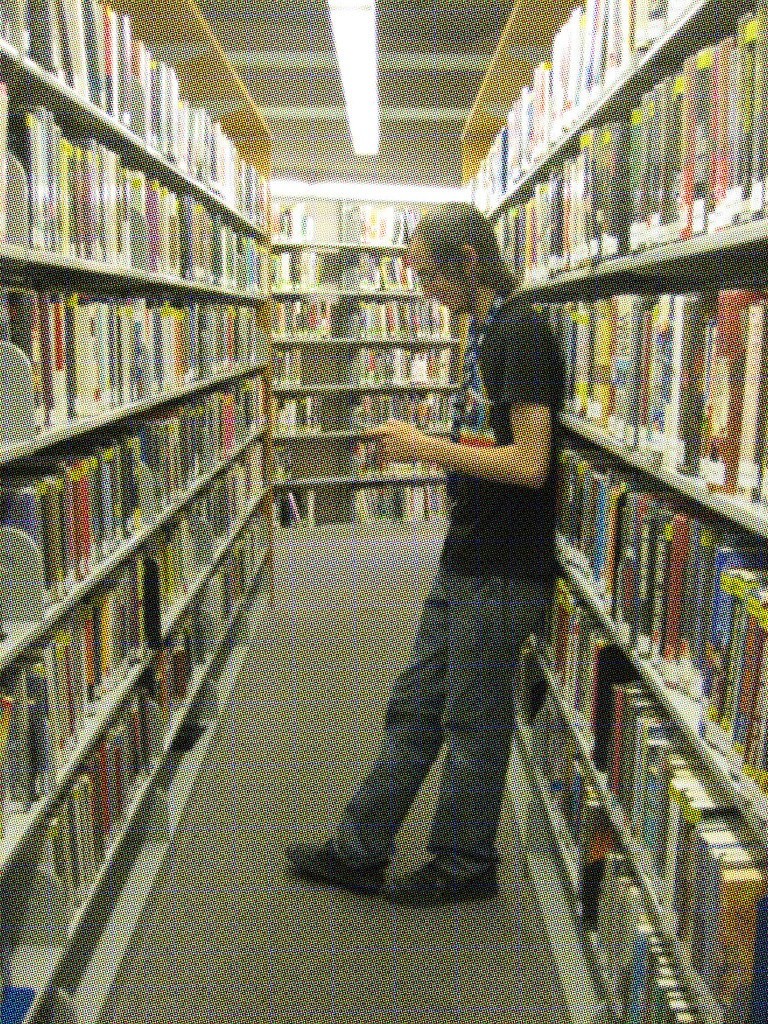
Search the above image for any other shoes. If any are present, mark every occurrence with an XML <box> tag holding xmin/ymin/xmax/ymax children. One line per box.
<box><xmin>285</xmin><ymin>842</ymin><xmax>387</xmax><ymax>892</ymax></box>
<box><xmin>386</xmin><ymin>859</ymin><xmax>504</xmax><ymax>904</ymax></box>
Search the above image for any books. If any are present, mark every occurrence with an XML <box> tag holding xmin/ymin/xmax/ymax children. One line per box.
<box><xmin>268</xmin><ymin>202</ymin><xmax>462</xmax><ymax>525</ymax></box>
<box><xmin>462</xmin><ymin>0</ymin><xmax>768</xmax><ymax>1024</ymax></box>
<box><xmin>0</xmin><ymin>0</ymin><xmax>271</xmax><ymax>921</ymax></box>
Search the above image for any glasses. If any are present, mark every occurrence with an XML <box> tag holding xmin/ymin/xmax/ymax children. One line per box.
<box><xmin>416</xmin><ymin>246</ymin><xmax>459</xmax><ymax>287</ymax></box>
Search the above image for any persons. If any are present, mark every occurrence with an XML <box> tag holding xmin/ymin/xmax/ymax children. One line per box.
<box><xmin>284</xmin><ymin>200</ymin><xmax>568</xmax><ymax>898</ymax></box>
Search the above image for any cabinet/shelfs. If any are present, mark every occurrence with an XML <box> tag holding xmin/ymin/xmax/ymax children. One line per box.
<box><xmin>0</xmin><ymin>0</ymin><xmax>768</xmax><ymax>1024</ymax></box>
